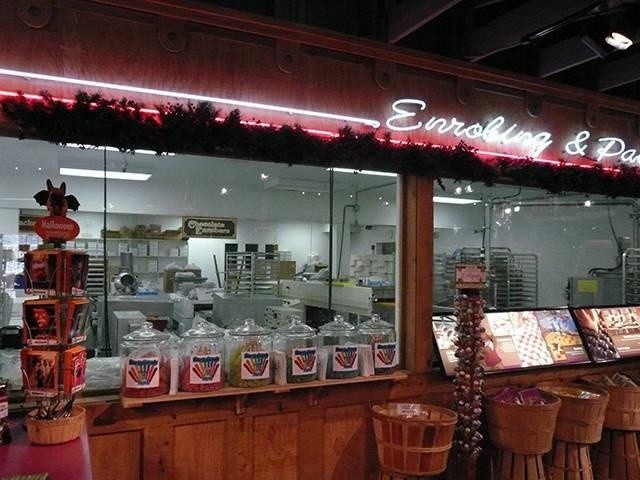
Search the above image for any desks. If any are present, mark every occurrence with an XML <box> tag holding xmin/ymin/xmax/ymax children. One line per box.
<box><xmin>0</xmin><ymin>420</ymin><xmax>92</xmax><ymax>480</ymax></box>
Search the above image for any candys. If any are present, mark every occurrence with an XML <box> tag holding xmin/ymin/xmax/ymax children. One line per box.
<box><xmin>287</xmin><ymin>356</ymin><xmax>318</xmax><ymax>383</ymax></box>
<box><xmin>452</xmin><ymin>294</ymin><xmax>486</xmax><ymax>464</ymax></box>
<box><xmin>372</xmin><ymin>347</ymin><xmax>394</xmax><ymax>375</ymax></box>
<box><xmin>327</xmin><ymin>352</ymin><xmax>360</xmax><ymax>379</ymax></box>
<box><xmin>228</xmin><ymin>342</ymin><xmax>273</xmax><ymax>387</ymax></box>
<box><xmin>121</xmin><ymin>352</ymin><xmax>171</xmax><ymax>397</ymax></box>
<box><xmin>178</xmin><ymin>346</ymin><xmax>225</xmax><ymax>392</ymax></box>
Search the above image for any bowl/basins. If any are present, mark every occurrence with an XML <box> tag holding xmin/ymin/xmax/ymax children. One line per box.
<box><xmin>114</xmin><ymin>271</ymin><xmax>139</xmax><ymax>295</ymax></box>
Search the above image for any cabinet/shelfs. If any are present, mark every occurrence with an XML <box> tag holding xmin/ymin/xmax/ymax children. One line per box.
<box><xmin>106</xmin><ymin>238</ymin><xmax>188</xmax><ymax>294</ymax></box>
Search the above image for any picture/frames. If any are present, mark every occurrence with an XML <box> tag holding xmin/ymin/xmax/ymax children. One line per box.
<box><xmin>20</xmin><ymin>249</ymin><xmax>92</xmax><ymax>398</ymax></box>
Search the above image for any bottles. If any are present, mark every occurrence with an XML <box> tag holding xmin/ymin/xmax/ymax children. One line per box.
<box><xmin>121</xmin><ymin>321</ymin><xmax>171</xmax><ymax>397</ymax></box>
<box><xmin>0</xmin><ymin>377</ymin><xmax>12</xmax><ymax>447</ymax></box>
<box><xmin>273</xmin><ymin>316</ymin><xmax>317</xmax><ymax>383</ymax></box>
<box><xmin>178</xmin><ymin>322</ymin><xmax>225</xmax><ymax>392</ymax></box>
<box><xmin>227</xmin><ymin>319</ymin><xmax>273</xmax><ymax>387</ymax></box>
<box><xmin>318</xmin><ymin>315</ymin><xmax>360</xmax><ymax>378</ymax></box>
<box><xmin>355</xmin><ymin>314</ymin><xmax>398</xmax><ymax>375</ymax></box>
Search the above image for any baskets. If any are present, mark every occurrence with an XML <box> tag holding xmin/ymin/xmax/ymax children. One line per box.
<box><xmin>26</xmin><ymin>402</ymin><xmax>86</xmax><ymax>444</ymax></box>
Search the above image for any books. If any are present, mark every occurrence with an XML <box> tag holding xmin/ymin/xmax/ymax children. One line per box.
<box><xmin>20</xmin><ymin>247</ymin><xmax>90</xmax><ymax>400</ymax></box>
